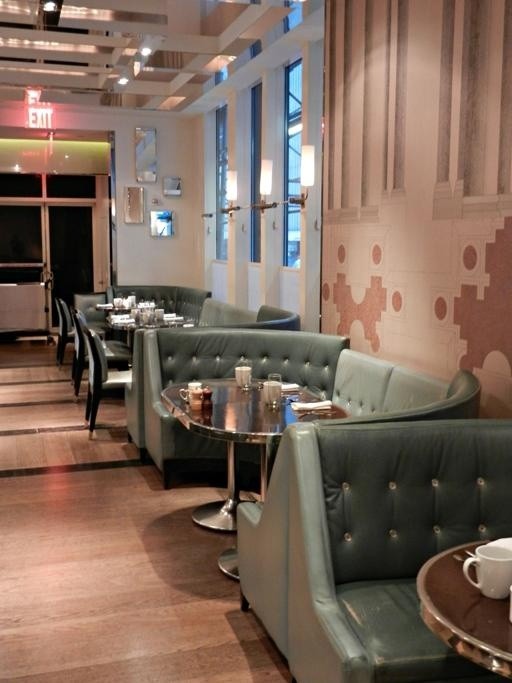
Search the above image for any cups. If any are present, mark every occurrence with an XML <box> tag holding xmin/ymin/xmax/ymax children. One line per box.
<box><xmin>263</xmin><ymin>373</ymin><xmax>282</xmax><ymax>410</ymax></box>
<box><xmin>234</xmin><ymin>366</ymin><xmax>252</xmax><ymax>387</ymax></box>
<box><xmin>178</xmin><ymin>382</ymin><xmax>202</xmax><ymax>402</ymax></box>
<box><xmin>462</xmin><ymin>544</ymin><xmax>512</xmax><ymax>600</ymax></box>
<box><xmin>114</xmin><ymin>294</ymin><xmax>164</xmax><ymax>325</ymax></box>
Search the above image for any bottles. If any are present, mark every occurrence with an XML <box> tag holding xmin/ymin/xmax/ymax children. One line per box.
<box><xmin>190</xmin><ymin>386</ymin><xmax>213</xmax><ymax>412</ymax></box>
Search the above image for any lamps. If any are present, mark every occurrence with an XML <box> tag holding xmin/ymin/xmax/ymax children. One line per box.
<box><xmin>254</xmin><ymin>159</ymin><xmax>277</xmax><ymax>209</ymax></box>
<box><xmin>221</xmin><ymin>168</ymin><xmax>242</xmax><ymax>215</ymax></box>
<box><xmin>290</xmin><ymin>144</ymin><xmax>318</xmax><ymax>207</ymax></box>
